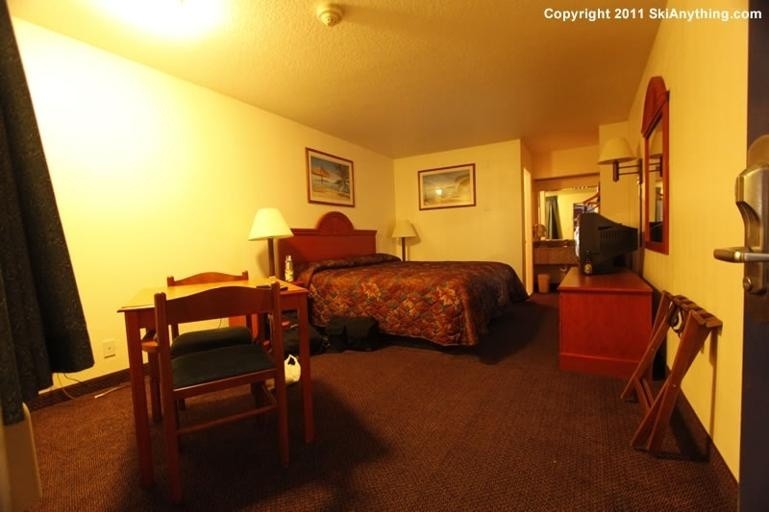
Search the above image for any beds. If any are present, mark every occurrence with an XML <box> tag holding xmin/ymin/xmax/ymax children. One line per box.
<box><xmin>277</xmin><ymin>211</ymin><xmax>532</xmax><ymax>353</ymax></box>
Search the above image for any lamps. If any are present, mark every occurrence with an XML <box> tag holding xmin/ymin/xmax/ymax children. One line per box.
<box><xmin>649</xmin><ymin>128</ymin><xmax>663</xmax><ymax>177</ymax></box>
<box><xmin>597</xmin><ymin>136</ymin><xmax>642</xmax><ymax>184</ymax></box>
<box><xmin>392</xmin><ymin>220</ymin><xmax>416</xmax><ymax>261</ymax></box>
<box><xmin>249</xmin><ymin>208</ymin><xmax>294</xmax><ymax>280</ymax></box>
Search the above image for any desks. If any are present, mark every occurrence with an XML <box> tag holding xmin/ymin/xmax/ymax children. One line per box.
<box><xmin>118</xmin><ymin>277</ymin><xmax>317</xmax><ymax>489</ymax></box>
<box><xmin>557</xmin><ymin>267</ymin><xmax>654</xmax><ymax>381</ymax></box>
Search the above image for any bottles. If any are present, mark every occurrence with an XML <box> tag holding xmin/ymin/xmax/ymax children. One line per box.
<box><xmin>284</xmin><ymin>255</ymin><xmax>294</xmax><ymax>284</ymax></box>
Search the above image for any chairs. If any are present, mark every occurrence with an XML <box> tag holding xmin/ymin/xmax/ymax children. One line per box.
<box><xmin>167</xmin><ymin>271</ymin><xmax>269</xmax><ymax>427</ymax></box>
<box><xmin>154</xmin><ymin>281</ymin><xmax>291</xmax><ymax>505</ymax></box>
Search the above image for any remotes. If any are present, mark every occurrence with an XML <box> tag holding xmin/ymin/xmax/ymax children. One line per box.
<box><xmin>256</xmin><ymin>283</ymin><xmax>288</xmax><ymax>291</ymax></box>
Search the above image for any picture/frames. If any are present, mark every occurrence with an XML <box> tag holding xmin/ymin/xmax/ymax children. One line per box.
<box><xmin>418</xmin><ymin>163</ymin><xmax>476</xmax><ymax>210</ymax></box>
<box><xmin>305</xmin><ymin>147</ymin><xmax>355</xmax><ymax>207</ymax></box>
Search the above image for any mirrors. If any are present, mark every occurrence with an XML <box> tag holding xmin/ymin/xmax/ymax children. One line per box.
<box><xmin>641</xmin><ymin>75</ymin><xmax>669</xmax><ymax>256</ymax></box>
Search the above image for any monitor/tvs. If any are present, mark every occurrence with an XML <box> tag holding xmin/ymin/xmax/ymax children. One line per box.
<box><xmin>579</xmin><ymin>211</ymin><xmax>640</xmax><ymax>275</ymax></box>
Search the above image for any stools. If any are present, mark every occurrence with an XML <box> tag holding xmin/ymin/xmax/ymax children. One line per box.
<box><xmin>621</xmin><ymin>290</ymin><xmax>722</xmax><ymax>454</ymax></box>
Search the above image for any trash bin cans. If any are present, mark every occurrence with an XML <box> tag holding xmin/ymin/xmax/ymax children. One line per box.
<box><xmin>538</xmin><ymin>274</ymin><xmax>551</xmax><ymax>293</ymax></box>
